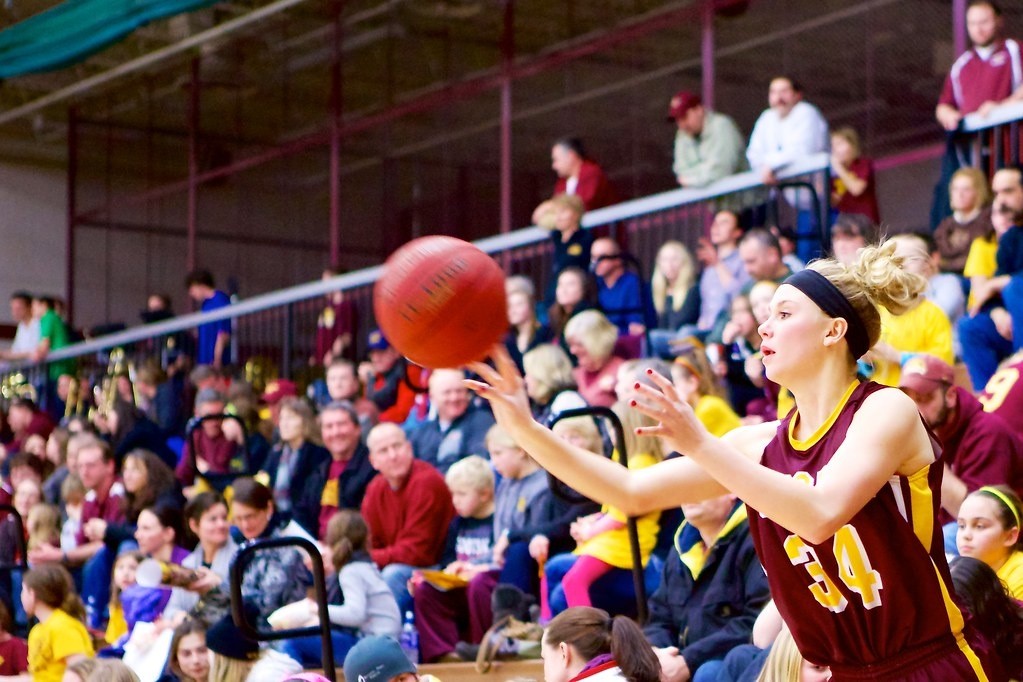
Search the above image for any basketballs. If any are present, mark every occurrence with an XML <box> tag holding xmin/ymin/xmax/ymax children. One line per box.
<box><xmin>374</xmin><ymin>236</ymin><xmax>508</xmax><ymax>365</ymax></box>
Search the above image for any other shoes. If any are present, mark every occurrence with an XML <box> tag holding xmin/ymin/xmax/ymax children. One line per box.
<box><xmin>454</xmin><ymin>640</ymin><xmax>474</xmax><ymax>661</ymax></box>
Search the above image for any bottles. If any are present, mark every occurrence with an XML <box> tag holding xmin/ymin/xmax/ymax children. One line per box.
<box><xmin>400</xmin><ymin>612</ymin><xmax>418</xmax><ymax>665</ymax></box>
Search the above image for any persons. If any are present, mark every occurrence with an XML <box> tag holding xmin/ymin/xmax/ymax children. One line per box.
<box><xmin>464</xmin><ymin>238</ymin><xmax>1009</xmax><ymax>681</ymax></box>
<box><xmin>746</xmin><ymin>74</ymin><xmax>835</xmax><ymax>266</ymax></box>
<box><xmin>826</xmin><ymin>128</ymin><xmax>883</xmax><ymax>242</ymax></box>
<box><xmin>934</xmin><ymin>1</ymin><xmax>1023</xmax><ymax>172</ymax></box>
<box><xmin>668</xmin><ymin>88</ymin><xmax>767</xmax><ymax>231</ymax></box>
<box><xmin>0</xmin><ymin>124</ymin><xmax>1023</xmax><ymax>681</ymax></box>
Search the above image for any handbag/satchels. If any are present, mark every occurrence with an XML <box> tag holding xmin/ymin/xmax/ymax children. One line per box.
<box><xmin>488</xmin><ymin>615</ymin><xmax>544</xmax><ymax>659</ymax></box>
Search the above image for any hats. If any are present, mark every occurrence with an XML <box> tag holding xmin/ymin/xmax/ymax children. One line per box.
<box><xmin>896</xmin><ymin>354</ymin><xmax>957</xmax><ymax>397</ymax></box>
<box><xmin>667</xmin><ymin>89</ymin><xmax>701</xmax><ymax>118</ymax></box>
<box><xmin>343</xmin><ymin>634</ymin><xmax>417</xmax><ymax>682</ymax></box>
<box><xmin>365</xmin><ymin>327</ymin><xmax>389</xmax><ymax>351</ymax></box>
<box><xmin>260</xmin><ymin>380</ymin><xmax>297</xmax><ymax>402</ymax></box>
<box><xmin>205</xmin><ymin>595</ymin><xmax>262</xmax><ymax>662</ymax></box>
